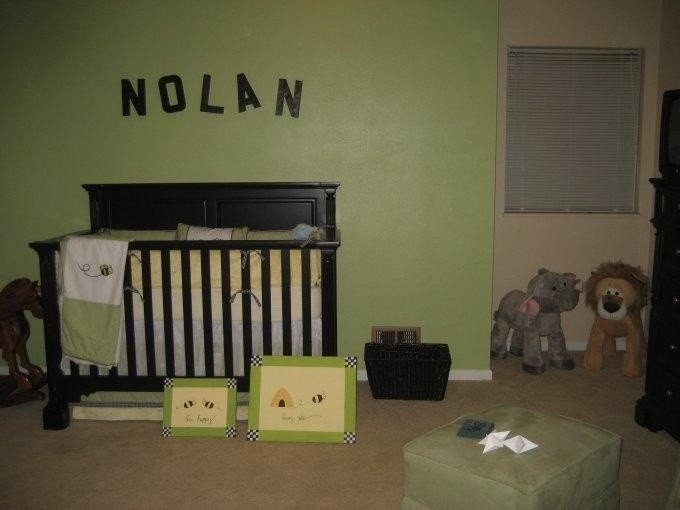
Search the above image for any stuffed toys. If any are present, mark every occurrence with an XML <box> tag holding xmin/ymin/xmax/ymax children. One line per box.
<box><xmin>490</xmin><ymin>268</ymin><xmax>582</xmax><ymax>375</ymax></box>
<box><xmin>582</xmin><ymin>261</ymin><xmax>647</xmax><ymax>378</ymax></box>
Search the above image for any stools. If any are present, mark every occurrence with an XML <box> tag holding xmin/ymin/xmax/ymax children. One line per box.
<box><xmin>402</xmin><ymin>404</ymin><xmax>623</xmax><ymax>509</ymax></box>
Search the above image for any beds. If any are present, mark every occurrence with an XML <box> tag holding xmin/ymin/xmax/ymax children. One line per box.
<box><xmin>28</xmin><ymin>181</ymin><xmax>341</xmax><ymax>432</ymax></box>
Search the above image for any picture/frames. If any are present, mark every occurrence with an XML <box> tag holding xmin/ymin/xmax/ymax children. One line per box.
<box><xmin>162</xmin><ymin>377</ymin><xmax>237</xmax><ymax>438</ymax></box>
<box><xmin>247</xmin><ymin>355</ymin><xmax>356</xmax><ymax>444</ymax></box>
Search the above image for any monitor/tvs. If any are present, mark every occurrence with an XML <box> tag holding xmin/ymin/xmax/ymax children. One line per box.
<box><xmin>659</xmin><ymin>89</ymin><xmax>680</xmax><ymax>182</ymax></box>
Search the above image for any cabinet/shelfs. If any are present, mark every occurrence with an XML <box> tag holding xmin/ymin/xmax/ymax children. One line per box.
<box><xmin>635</xmin><ymin>178</ymin><xmax>680</xmax><ymax>443</ymax></box>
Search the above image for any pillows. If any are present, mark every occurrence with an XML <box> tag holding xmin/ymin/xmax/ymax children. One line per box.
<box><xmin>174</xmin><ymin>223</ymin><xmax>249</xmax><ymax>240</ymax></box>
